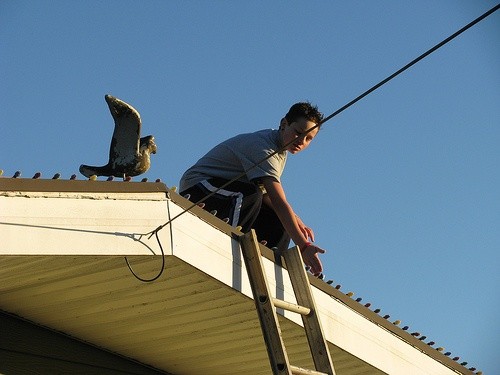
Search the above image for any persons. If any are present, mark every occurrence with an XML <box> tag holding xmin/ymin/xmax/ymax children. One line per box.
<box><xmin>179</xmin><ymin>102</ymin><xmax>326</xmax><ymax>277</ymax></box>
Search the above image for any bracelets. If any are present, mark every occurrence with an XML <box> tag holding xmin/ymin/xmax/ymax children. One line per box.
<box><xmin>301</xmin><ymin>241</ymin><xmax>311</xmax><ymax>255</ymax></box>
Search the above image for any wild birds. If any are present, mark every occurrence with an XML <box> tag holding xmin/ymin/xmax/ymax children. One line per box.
<box><xmin>77</xmin><ymin>92</ymin><xmax>158</xmax><ymax>179</ymax></box>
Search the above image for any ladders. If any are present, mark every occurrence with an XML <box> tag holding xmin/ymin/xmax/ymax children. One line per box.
<box><xmin>239</xmin><ymin>227</ymin><xmax>335</xmax><ymax>375</ymax></box>
